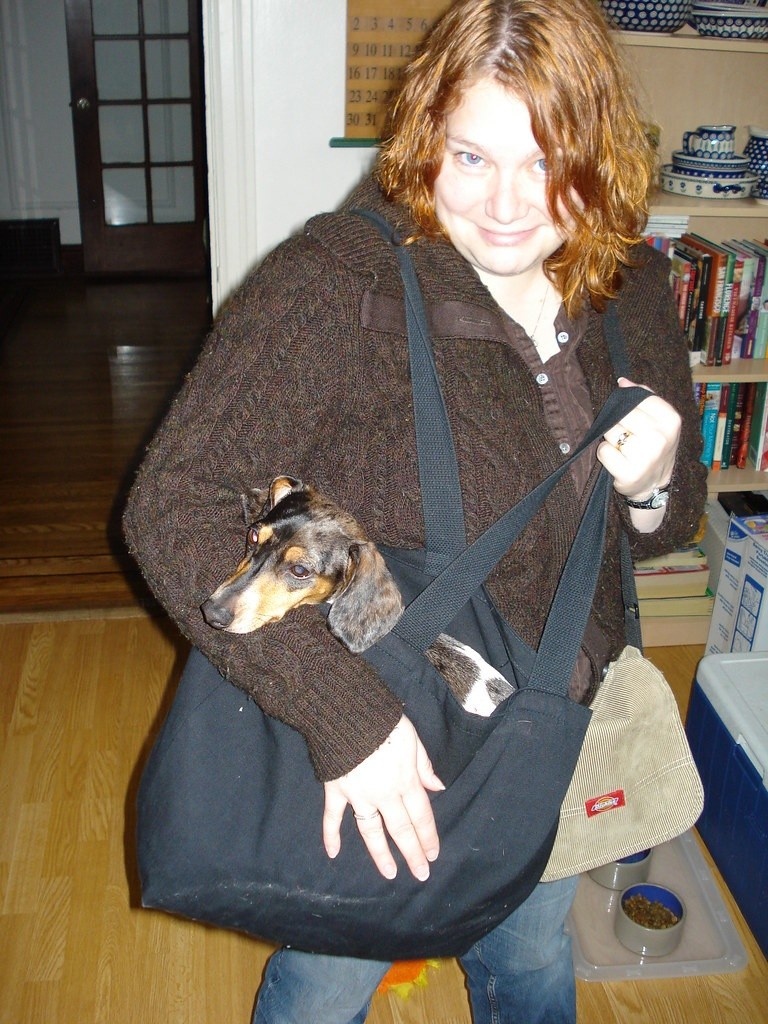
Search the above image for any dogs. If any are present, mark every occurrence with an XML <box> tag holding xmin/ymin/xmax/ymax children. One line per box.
<box><xmin>202</xmin><ymin>475</ymin><xmax>515</xmax><ymax>720</ymax></box>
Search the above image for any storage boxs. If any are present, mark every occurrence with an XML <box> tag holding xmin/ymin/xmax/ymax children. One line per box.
<box><xmin>701</xmin><ymin>489</ymin><xmax>768</xmax><ymax>592</ymax></box>
<box><xmin>684</xmin><ymin>650</ymin><xmax>768</xmax><ymax>960</ymax></box>
<box><xmin>704</xmin><ymin>515</ymin><xmax>768</xmax><ymax>655</ymax></box>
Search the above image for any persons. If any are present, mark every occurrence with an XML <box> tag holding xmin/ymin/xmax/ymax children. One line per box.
<box><xmin>122</xmin><ymin>0</ymin><xmax>708</xmax><ymax>1024</ymax></box>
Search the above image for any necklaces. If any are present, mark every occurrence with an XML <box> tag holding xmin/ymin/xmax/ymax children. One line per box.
<box><xmin>528</xmin><ymin>280</ymin><xmax>550</xmax><ymax>348</ymax></box>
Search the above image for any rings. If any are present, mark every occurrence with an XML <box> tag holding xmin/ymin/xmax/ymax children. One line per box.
<box><xmin>616</xmin><ymin>431</ymin><xmax>633</xmax><ymax>451</ymax></box>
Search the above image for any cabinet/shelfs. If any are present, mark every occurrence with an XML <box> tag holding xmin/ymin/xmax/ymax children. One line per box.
<box><xmin>611</xmin><ymin>30</ymin><xmax>768</xmax><ymax>492</ymax></box>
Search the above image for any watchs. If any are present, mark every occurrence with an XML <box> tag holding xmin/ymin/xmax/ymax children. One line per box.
<box><xmin>627</xmin><ymin>478</ymin><xmax>670</xmax><ymax>510</ymax></box>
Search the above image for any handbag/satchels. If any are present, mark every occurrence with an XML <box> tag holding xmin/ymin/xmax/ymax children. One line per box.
<box><xmin>530</xmin><ymin>645</ymin><xmax>705</xmax><ymax>886</ymax></box>
<box><xmin>136</xmin><ymin>208</ymin><xmax>652</xmax><ymax>962</ymax></box>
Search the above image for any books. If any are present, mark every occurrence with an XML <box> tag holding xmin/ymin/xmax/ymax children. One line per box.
<box><xmin>693</xmin><ymin>382</ymin><xmax>768</xmax><ymax>476</ymax></box>
<box><xmin>633</xmin><ymin>547</ymin><xmax>715</xmax><ymax>617</ymax></box>
<box><xmin>640</xmin><ymin>215</ymin><xmax>768</xmax><ymax>368</ymax></box>
